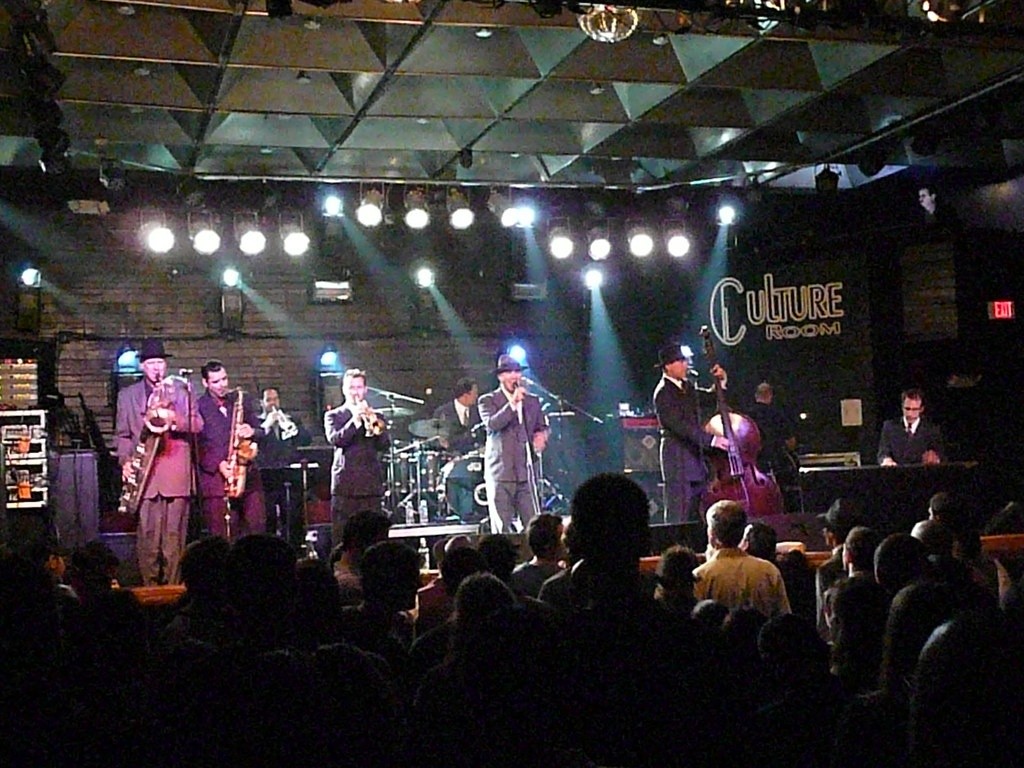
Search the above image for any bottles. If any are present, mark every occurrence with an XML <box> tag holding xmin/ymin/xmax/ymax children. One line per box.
<box><xmin>419</xmin><ymin>499</ymin><xmax>429</xmax><ymax>524</ymax></box>
<box><xmin>405</xmin><ymin>501</ymin><xmax>415</xmax><ymax>525</ymax></box>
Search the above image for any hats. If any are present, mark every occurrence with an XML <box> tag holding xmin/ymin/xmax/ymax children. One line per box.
<box><xmin>489</xmin><ymin>355</ymin><xmax>530</xmax><ymax>374</ymax></box>
<box><xmin>135</xmin><ymin>340</ymin><xmax>174</xmax><ymax>363</ymax></box>
<box><xmin>653</xmin><ymin>344</ymin><xmax>687</xmax><ymax>368</ymax></box>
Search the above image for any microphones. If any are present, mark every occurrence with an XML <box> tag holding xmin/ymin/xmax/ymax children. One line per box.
<box><xmin>513</xmin><ymin>382</ymin><xmax>525</xmax><ymax>397</ymax></box>
<box><xmin>521</xmin><ymin>377</ymin><xmax>534</xmax><ymax>386</ymax></box>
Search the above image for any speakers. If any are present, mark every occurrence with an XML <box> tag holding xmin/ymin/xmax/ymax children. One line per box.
<box><xmin>612</xmin><ymin>415</ymin><xmax>662</xmax><ymax>474</ymax></box>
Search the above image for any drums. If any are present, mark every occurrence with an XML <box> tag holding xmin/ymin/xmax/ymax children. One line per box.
<box><xmin>392</xmin><ymin>451</ymin><xmax>490</xmax><ymax>525</ymax></box>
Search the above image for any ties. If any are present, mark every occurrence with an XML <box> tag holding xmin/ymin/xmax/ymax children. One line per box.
<box><xmin>906</xmin><ymin>423</ymin><xmax>913</xmax><ymax>436</ymax></box>
<box><xmin>463</xmin><ymin>409</ymin><xmax>469</xmax><ymax>428</ymax></box>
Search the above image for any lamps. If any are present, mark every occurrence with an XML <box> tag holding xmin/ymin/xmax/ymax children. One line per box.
<box><xmin>660</xmin><ymin>216</ymin><xmax>696</xmax><ymax>258</ymax></box>
<box><xmin>186</xmin><ymin>211</ymin><xmax>222</xmax><ymax>256</ymax></box>
<box><xmin>445</xmin><ymin>188</ymin><xmax>475</xmax><ymax>231</ymax></box>
<box><xmin>486</xmin><ymin>192</ymin><xmax>520</xmax><ymax>229</ymax></box>
<box><xmin>625</xmin><ymin>217</ymin><xmax>662</xmax><ymax>259</ymax></box>
<box><xmin>545</xmin><ymin>215</ymin><xmax>578</xmax><ymax>262</ymax></box>
<box><xmin>232</xmin><ymin>210</ymin><xmax>266</xmax><ymax>257</ymax></box>
<box><xmin>576</xmin><ymin>5</ymin><xmax>639</xmax><ymax>45</ymax></box>
<box><xmin>354</xmin><ymin>182</ymin><xmax>386</xmax><ymax>227</ymax></box>
<box><xmin>400</xmin><ymin>186</ymin><xmax>433</xmax><ymax>231</ymax></box>
<box><xmin>583</xmin><ymin>216</ymin><xmax>615</xmax><ymax>262</ymax></box>
<box><xmin>138</xmin><ymin>210</ymin><xmax>176</xmax><ymax>254</ymax></box>
<box><xmin>277</xmin><ymin>211</ymin><xmax>311</xmax><ymax>256</ymax></box>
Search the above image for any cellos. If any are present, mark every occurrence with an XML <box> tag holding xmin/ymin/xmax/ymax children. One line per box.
<box><xmin>696</xmin><ymin>323</ymin><xmax>785</xmax><ymax>531</ymax></box>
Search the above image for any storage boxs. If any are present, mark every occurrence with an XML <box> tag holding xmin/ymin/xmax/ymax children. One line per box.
<box><xmin>6</xmin><ymin>458</ymin><xmax>48</xmax><ymax>509</ymax></box>
<box><xmin>0</xmin><ymin>409</ymin><xmax>48</xmax><ymax>460</ymax></box>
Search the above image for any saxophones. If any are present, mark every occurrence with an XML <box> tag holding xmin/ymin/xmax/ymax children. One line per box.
<box><xmin>270</xmin><ymin>405</ymin><xmax>300</xmax><ymax>441</ymax></box>
<box><xmin>116</xmin><ymin>373</ymin><xmax>176</xmax><ymax>516</ymax></box>
<box><xmin>223</xmin><ymin>384</ymin><xmax>259</xmax><ymax>499</ymax></box>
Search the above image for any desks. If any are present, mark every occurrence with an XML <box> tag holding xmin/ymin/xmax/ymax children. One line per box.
<box><xmin>798</xmin><ymin>452</ymin><xmax>861</xmax><ymax>469</ymax></box>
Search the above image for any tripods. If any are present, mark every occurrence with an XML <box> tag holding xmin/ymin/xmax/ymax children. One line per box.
<box><xmin>388</xmin><ymin>434</ymin><xmax>445</xmax><ymax>524</ymax></box>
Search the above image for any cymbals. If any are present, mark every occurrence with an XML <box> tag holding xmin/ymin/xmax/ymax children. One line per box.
<box><xmin>373</xmin><ymin>406</ymin><xmax>416</xmax><ymax>420</ymax></box>
<box><xmin>408</xmin><ymin>417</ymin><xmax>458</xmax><ymax>439</ymax></box>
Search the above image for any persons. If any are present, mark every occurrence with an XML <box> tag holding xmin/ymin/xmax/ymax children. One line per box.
<box><xmin>324</xmin><ymin>370</ymin><xmax>390</xmax><ymax>548</ymax></box>
<box><xmin>477</xmin><ymin>352</ymin><xmax>551</xmax><ymax>534</ymax></box>
<box><xmin>115</xmin><ymin>339</ymin><xmax>204</xmax><ymax>585</ymax></box>
<box><xmin>255</xmin><ymin>388</ymin><xmax>313</xmax><ymax>541</ymax></box>
<box><xmin>652</xmin><ymin>343</ymin><xmax>728</xmax><ymax>524</ymax></box>
<box><xmin>0</xmin><ymin>472</ymin><xmax>1024</xmax><ymax>768</ymax></box>
<box><xmin>434</xmin><ymin>375</ymin><xmax>485</xmax><ymax>522</ymax></box>
<box><xmin>746</xmin><ymin>382</ymin><xmax>796</xmax><ymax>474</ymax></box>
<box><xmin>879</xmin><ymin>388</ymin><xmax>944</xmax><ymax>467</ymax></box>
<box><xmin>194</xmin><ymin>362</ymin><xmax>266</xmax><ymax>542</ymax></box>
